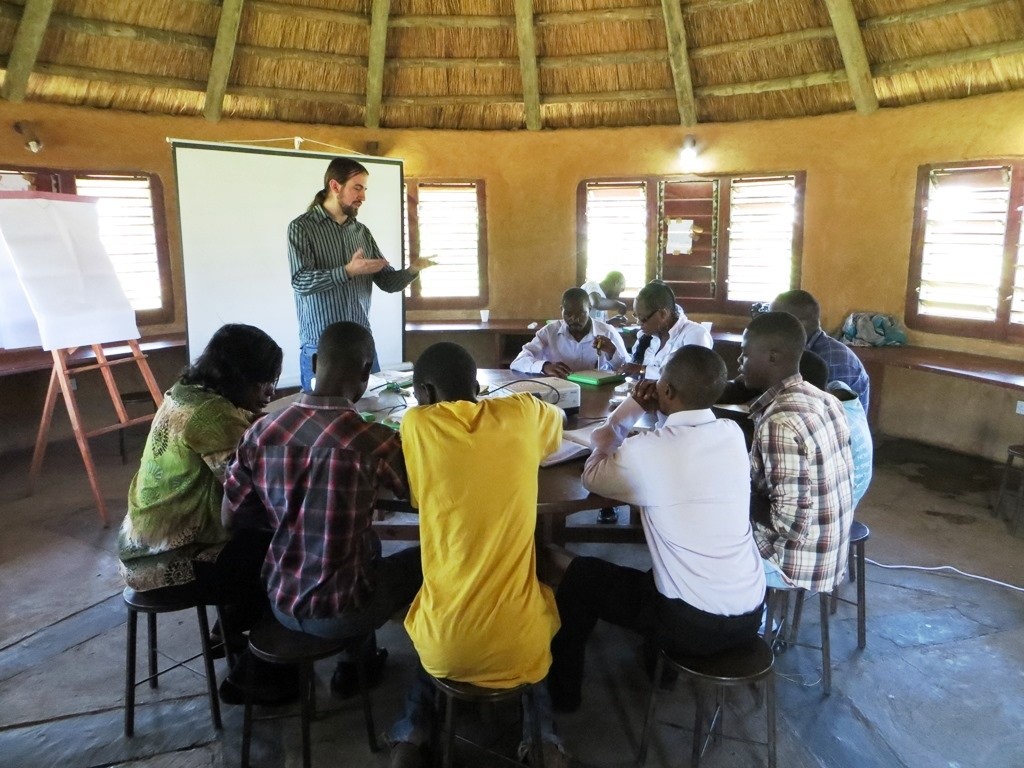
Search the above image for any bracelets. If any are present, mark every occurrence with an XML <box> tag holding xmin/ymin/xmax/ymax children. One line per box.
<box><xmin>639</xmin><ymin>365</ymin><xmax>647</xmax><ymax>375</ymax></box>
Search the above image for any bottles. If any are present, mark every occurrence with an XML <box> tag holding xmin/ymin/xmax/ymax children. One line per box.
<box><xmin>751</xmin><ymin>303</ymin><xmax>769</xmax><ymax>321</ymax></box>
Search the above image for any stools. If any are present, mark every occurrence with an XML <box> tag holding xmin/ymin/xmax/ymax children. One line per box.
<box><xmin>992</xmin><ymin>444</ymin><xmax>1024</xmax><ymax>538</ymax></box>
<box><xmin>120</xmin><ymin>391</ymin><xmax>164</xmax><ymax>466</ymax></box>
<box><xmin>123</xmin><ymin>516</ymin><xmax>870</xmax><ymax>768</ymax></box>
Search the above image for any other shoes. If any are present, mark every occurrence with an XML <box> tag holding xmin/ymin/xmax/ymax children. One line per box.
<box><xmin>204</xmin><ymin>633</ymin><xmax>251</xmax><ymax>659</ymax></box>
<box><xmin>638</xmin><ymin>643</ymin><xmax>678</xmax><ymax>688</ymax></box>
<box><xmin>597</xmin><ymin>506</ymin><xmax>617</xmax><ymax>523</ymax></box>
<box><xmin>220</xmin><ymin>662</ymin><xmax>299</xmax><ymax>706</ymax></box>
<box><xmin>391</xmin><ymin>742</ymin><xmax>425</xmax><ymax>768</ymax></box>
<box><xmin>331</xmin><ymin>647</ymin><xmax>388</xmax><ymax>697</ymax></box>
<box><xmin>545</xmin><ymin>679</ymin><xmax>581</xmax><ymax>712</ymax></box>
<box><xmin>526</xmin><ymin>740</ymin><xmax>565</xmax><ymax>768</ymax></box>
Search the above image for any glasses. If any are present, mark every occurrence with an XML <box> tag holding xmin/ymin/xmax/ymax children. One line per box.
<box><xmin>632</xmin><ymin>308</ymin><xmax>661</xmax><ymax>323</ymax></box>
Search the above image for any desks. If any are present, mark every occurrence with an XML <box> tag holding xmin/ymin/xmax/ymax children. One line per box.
<box><xmin>0</xmin><ymin>319</ymin><xmax>1024</xmax><ymax>470</ymax></box>
<box><xmin>298</xmin><ymin>368</ymin><xmax>667</xmax><ymax>583</ymax></box>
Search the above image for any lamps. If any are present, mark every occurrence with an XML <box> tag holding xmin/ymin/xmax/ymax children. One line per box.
<box><xmin>14</xmin><ymin>119</ymin><xmax>42</xmax><ymax>153</ymax></box>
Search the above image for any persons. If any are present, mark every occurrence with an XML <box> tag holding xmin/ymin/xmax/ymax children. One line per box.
<box><xmin>581</xmin><ymin>272</ymin><xmax>627</xmax><ymax>323</ymax></box>
<box><xmin>738</xmin><ymin>290</ymin><xmax>873</xmax><ymax>631</ymax></box>
<box><xmin>616</xmin><ymin>280</ymin><xmax>713</xmax><ymax>380</ymax></box>
<box><xmin>287</xmin><ymin>157</ymin><xmax>439</xmax><ymax>394</ymax></box>
<box><xmin>510</xmin><ymin>287</ymin><xmax>630</xmax><ymax>523</ymax></box>
<box><xmin>222</xmin><ymin>321</ymin><xmax>424</xmax><ymax>702</ymax></box>
<box><xmin>382</xmin><ymin>342</ymin><xmax>564</xmax><ymax>768</ymax></box>
<box><xmin>119</xmin><ymin>325</ymin><xmax>282</xmax><ymax>705</ymax></box>
<box><xmin>546</xmin><ymin>345</ymin><xmax>766</xmax><ymax>714</ymax></box>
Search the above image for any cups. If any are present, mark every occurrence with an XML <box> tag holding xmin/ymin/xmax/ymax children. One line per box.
<box><xmin>480</xmin><ymin>310</ymin><xmax>489</xmax><ymax>322</ymax></box>
